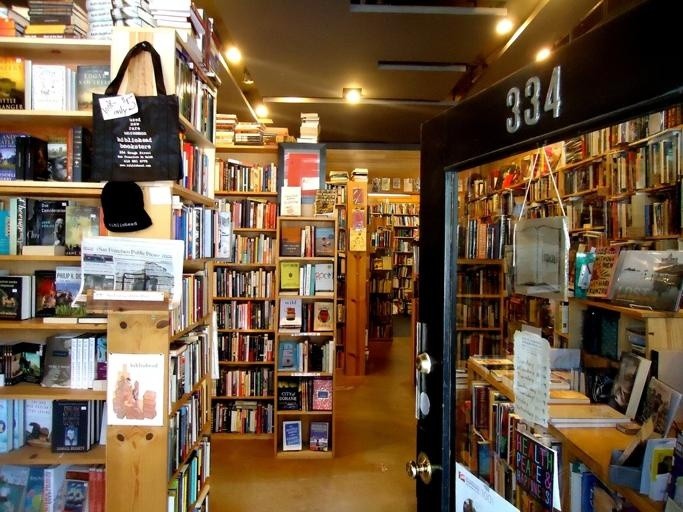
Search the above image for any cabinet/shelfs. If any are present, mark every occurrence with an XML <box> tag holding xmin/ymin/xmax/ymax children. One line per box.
<box><xmin>274</xmin><ymin>216</ymin><xmax>338</xmax><ymax>459</ymax></box>
<box><xmin>2</xmin><ymin>0</ymin><xmax>216</xmax><ymax>511</ymax></box>
<box><xmin>3</xmin><ymin>0</ymin><xmax>683</xmax><ymax>511</ymax></box>
<box><xmin>454</xmin><ymin>105</ymin><xmax>683</xmax><ymax>510</ymax></box>
<box><xmin>217</xmin><ymin>144</ymin><xmax>276</xmax><ymax>440</ymax></box>
<box><xmin>327</xmin><ymin>182</ymin><xmax>419</xmax><ymax>375</ymax></box>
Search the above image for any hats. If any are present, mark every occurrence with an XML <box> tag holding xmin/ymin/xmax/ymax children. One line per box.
<box><xmin>98</xmin><ymin>179</ymin><xmax>153</xmax><ymax>233</ymax></box>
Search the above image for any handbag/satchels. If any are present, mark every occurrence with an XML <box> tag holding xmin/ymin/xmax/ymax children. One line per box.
<box><xmin>86</xmin><ymin>40</ymin><xmax>185</xmax><ymax>183</ymax></box>
